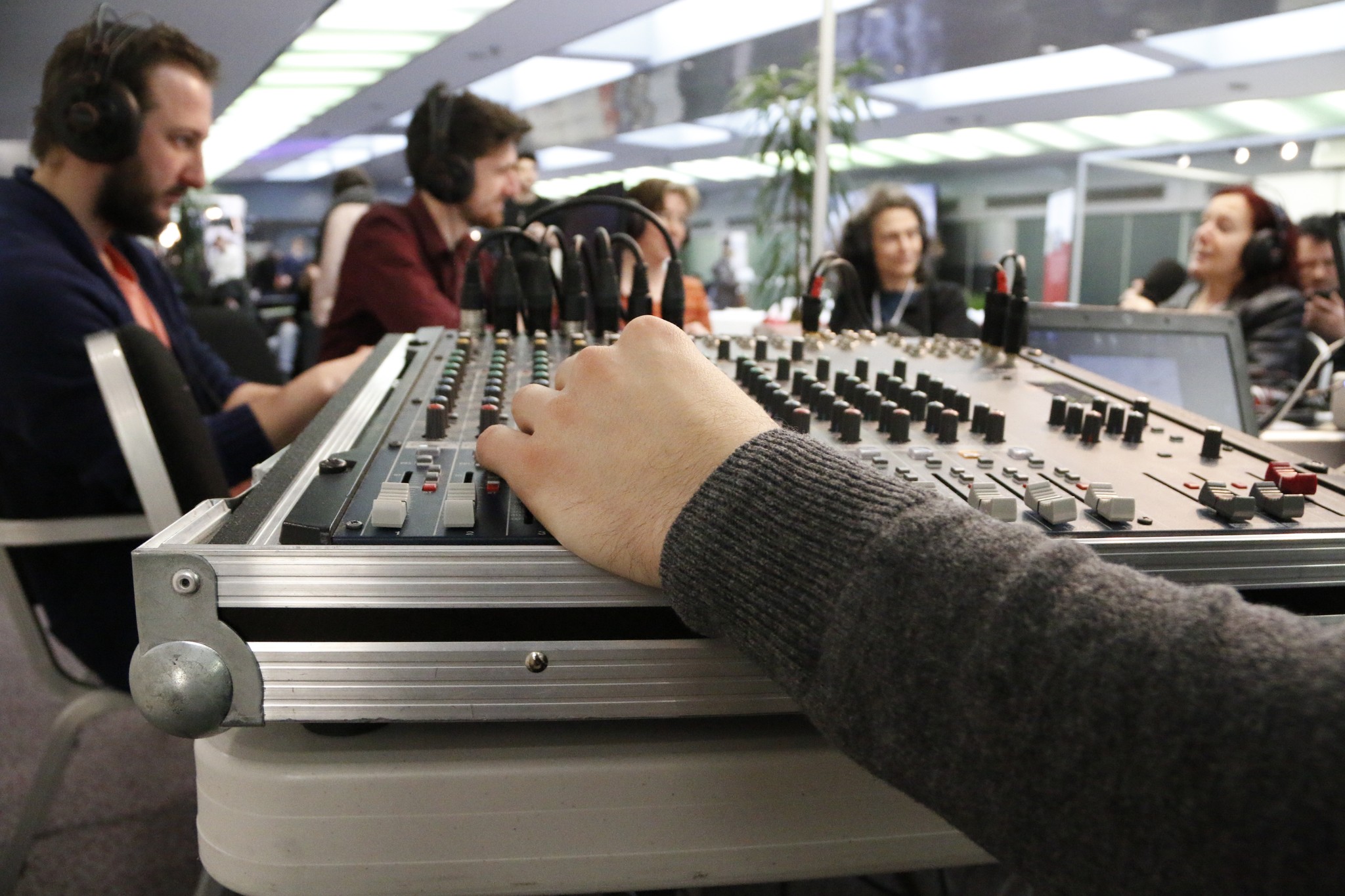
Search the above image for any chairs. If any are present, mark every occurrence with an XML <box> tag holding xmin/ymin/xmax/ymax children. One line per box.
<box><xmin>0</xmin><ymin>311</ymin><xmax>283</xmax><ymax>896</ymax></box>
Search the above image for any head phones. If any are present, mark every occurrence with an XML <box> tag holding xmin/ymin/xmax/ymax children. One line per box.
<box><xmin>418</xmin><ymin>81</ymin><xmax>475</xmax><ymax>206</ymax></box>
<box><xmin>1239</xmin><ymin>200</ymin><xmax>1291</xmax><ymax>279</ymax></box>
<box><xmin>843</xmin><ymin>199</ymin><xmax>928</xmax><ymax>266</ymax></box>
<box><xmin>57</xmin><ymin>0</ymin><xmax>158</xmax><ymax>162</ymax></box>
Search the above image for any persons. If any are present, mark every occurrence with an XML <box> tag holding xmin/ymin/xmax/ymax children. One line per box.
<box><xmin>276</xmin><ymin>83</ymin><xmax>747</xmax><ymax>340</ymax></box>
<box><xmin>469</xmin><ymin>314</ymin><xmax>1345</xmax><ymax>896</ymax></box>
<box><xmin>827</xmin><ymin>184</ymin><xmax>984</xmax><ymax>341</ymax></box>
<box><xmin>1102</xmin><ymin>180</ymin><xmax>1345</xmax><ymax>410</ymax></box>
<box><xmin>0</xmin><ymin>3</ymin><xmax>379</xmax><ymax>695</ymax></box>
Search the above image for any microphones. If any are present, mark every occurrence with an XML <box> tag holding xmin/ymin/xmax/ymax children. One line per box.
<box><xmin>1140</xmin><ymin>258</ymin><xmax>1186</xmax><ymax>304</ymax></box>
